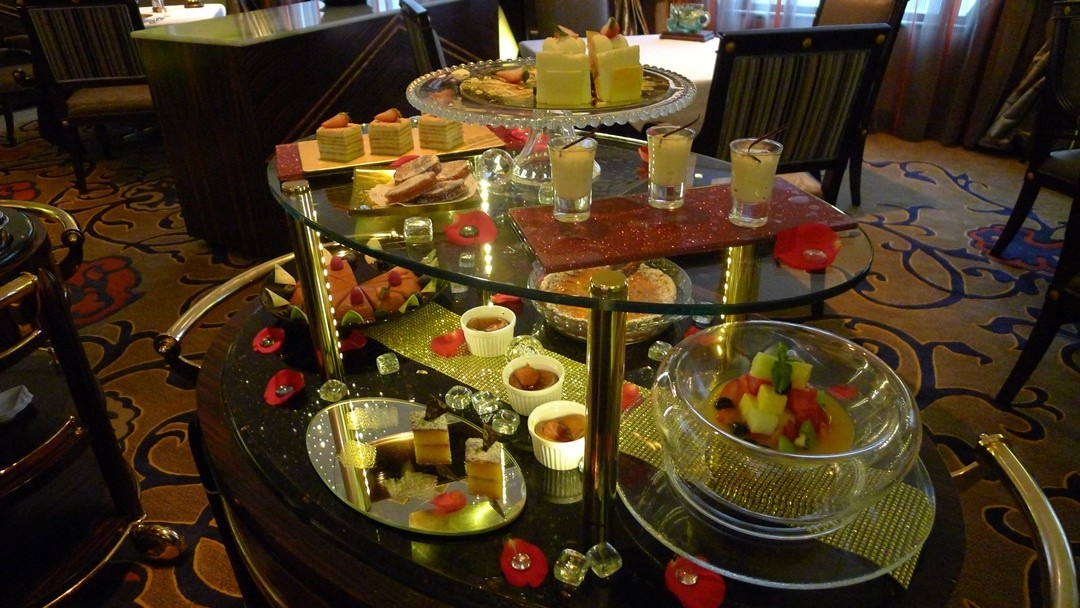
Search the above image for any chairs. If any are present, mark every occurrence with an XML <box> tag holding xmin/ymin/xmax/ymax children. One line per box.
<box><xmin>812</xmin><ymin>0</ymin><xmax>910</xmax><ymax>206</ymax></box>
<box><xmin>0</xmin><ymin>34</ymin><xmax>35</xmax><ymax>147</ymax></box>
<box><xmin>991</xmin><ymin>177</ymin><xmax>1080</xmax><ymax>406</ymax></box>
<box><xmin>988</xmin><ymin>0</ymin><xmax>1080</xmax><ymax>257</ymax></box>
<box><xmin>400</xmin><ymin>0</ymin><xmax>448</xmax><ymax>77</ymax></box>
<box><xmin>522</xmin><ymin>0</ymin><xmax>617</xmax><ymax>41</ymax></box>
<box><xmin>14</xmin><ymin>0</ymin><xmax>157</xmax><ymax>194</ymax></box>
<box><xmin>691</xmin><ymin>21</ymin><xmax>895</xmax><ymax>318</ymax></box>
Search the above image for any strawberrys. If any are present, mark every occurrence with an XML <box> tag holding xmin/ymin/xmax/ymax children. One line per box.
<box><xmin>495</xmin><ymin>66</ymin><xmax>529</xmax><ymax>84</ymax></box>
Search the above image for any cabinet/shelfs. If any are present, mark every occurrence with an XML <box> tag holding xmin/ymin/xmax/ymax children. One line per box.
<box><xmin>1</xmin><ymin>196</ymin><xmax>150</xmax><ymax>608</ymax></box>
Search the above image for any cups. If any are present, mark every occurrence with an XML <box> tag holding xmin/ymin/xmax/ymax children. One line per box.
<box><xmin>546</xmin><ymin>136</ymin><xmax>598</xmax><ymax>222</ymax></box>
<box><xmin>730</xmin><ymin>137</ymin><xmax>785</xmax><ymax>228</ymax></box>
<box><xmin>666</xmin><ymin>3</ymin><xmax>711</xmax><ymax>35</ymax></box>
<box><xmin>644</xmin><ymin>123</ymin><xmax>696</xmax><ymax>210</ymax></box>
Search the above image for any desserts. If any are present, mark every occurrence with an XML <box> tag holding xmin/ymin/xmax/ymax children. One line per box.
<box><xmin>410</xmin><ymin>394</ymin><xmax>450</xmax><ymax>446</ymax></box>
<box><xmin>291</xmin><ymin>245</ymin><xmax>422</xmax><ymax>319</ymax></box>
<box><xmin>316</xmin><ymin>106</ymin><xmax>464</xmax><ymax>163</ymax></box>
<box><xmin>464</xmin><ymin>422</ymin><xmax>503</xmax><ymax>480</ymax></box>
<box><xmin>414</xmin><ymin>446</ymin><xmax>461</xmax><ymax>482</ymax></box>
<box><xmin>467</xmin><ymin>476</ymin><xmax>506</xmax><ymax>520</ymax></box>
<box><xmin>535</xmin><ymin>16</ymin><xmax>643</xmax><ymax>104</ymax></box>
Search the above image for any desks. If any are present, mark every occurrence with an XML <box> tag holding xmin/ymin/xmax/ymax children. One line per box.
<box><xmin>518</xmin><ymin>30</ymin><xmax>723</xmax><ymax>144</ymax></box>
<box><xmin>128</xmin><ymin>0</ymin><xmax>500</xmax><ymax>255</ymax></box>
<box><xmin>139</xmin><ymin>4</ymin><xmax>227</xmax><ymax>27</ymax></box>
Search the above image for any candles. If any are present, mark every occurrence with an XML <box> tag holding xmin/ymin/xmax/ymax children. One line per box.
<box><xmin>190</xmin><ymin>113</ymin><xmax>975</xmax><ymax>608</ymax></box>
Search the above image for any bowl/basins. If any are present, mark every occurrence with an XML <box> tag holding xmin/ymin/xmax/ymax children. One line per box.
<box><xmin>651</xmin><ymin>324</ymin><xmax>925</xmax><ymax>542</ymax></box>
<box><xmin>459</xmin><ymin>304</ymin><xmax>518</xmax><ymax>357</ymax></box>
<box><xmin>502</xmin><ymin>354</ymin><xmax>566</xmax><ymax>416</ymax></box>
<box><xmin>525</xmin><ymin>399</ymin><xmax>588</xmax><ymax>471</ymax></box>
<box><xmin>528</xmin><ymin>250</ymin><xmax>694</xmax><ymax>347</ymax></box>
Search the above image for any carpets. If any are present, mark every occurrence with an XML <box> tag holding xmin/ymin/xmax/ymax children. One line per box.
<box><xmin>0</xmin><ymin>132</ymin><xmax>1080</xmax><ymax>608</ymax></box>
